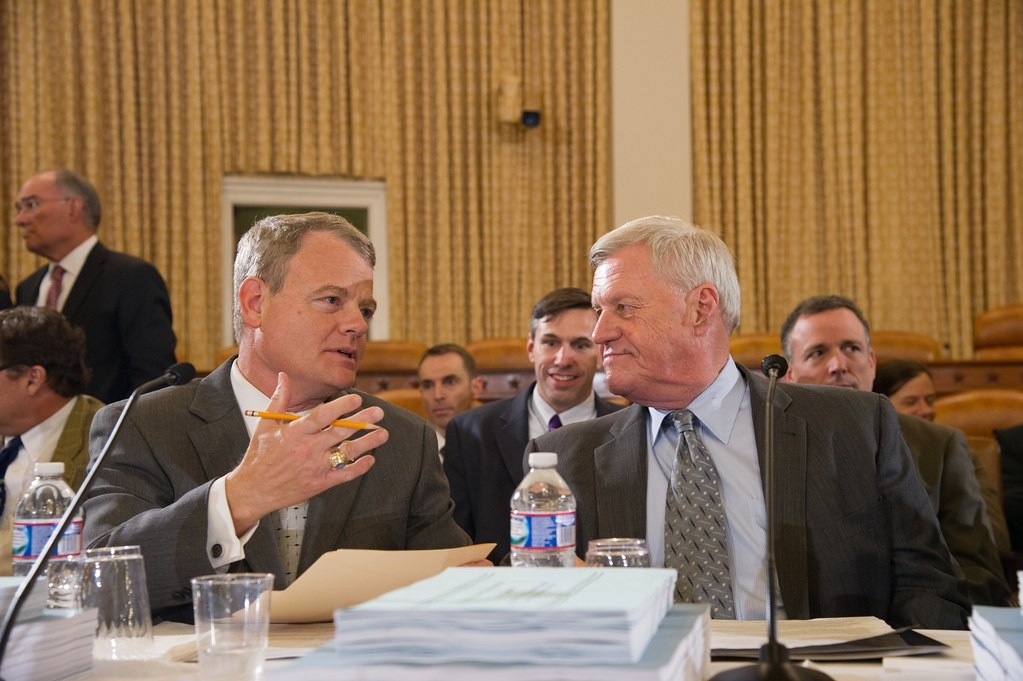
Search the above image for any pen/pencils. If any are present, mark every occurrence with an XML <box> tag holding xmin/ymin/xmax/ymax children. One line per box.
<box><xmin>245</xmin><ymin>406</ymin><xmax>384</xmax><ymax>432</ymax></box>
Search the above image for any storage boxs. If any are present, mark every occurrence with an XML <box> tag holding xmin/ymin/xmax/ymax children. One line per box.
<box><xmin>351</xmin><ymin>339</ymin><xmax>429</xmax><ymax>370</ymax></box>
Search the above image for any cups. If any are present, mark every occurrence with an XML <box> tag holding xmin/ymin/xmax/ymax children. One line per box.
<box><xmin>82</xmin><ymin>545</ymin><xmax>153</xmax><ymax>654</ymax></box>
<box><xmin>586</xmin><ymin>538</ymin><xmax>651</xmax><ymax>567</ymax></box>
<box><xmin>190</xmin><ymin>573</ymin><xmax>274</xmax><ymax>671</ymax></box>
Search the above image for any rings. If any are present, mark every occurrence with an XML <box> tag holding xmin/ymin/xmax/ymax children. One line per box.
<box><xmin>328</xmin><ymin>445</ymin><xmax>348</xmax><ymax>469</ymax></box>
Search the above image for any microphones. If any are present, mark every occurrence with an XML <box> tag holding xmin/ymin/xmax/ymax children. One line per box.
<box><xmin>0</xmin><ymin>362</ymin><xmax>197</xmax><ymax>681</ymax></box>
<box><xmin>709</xmin><ymin>353</ymin><xmax>835</xmax><ymax>681</ymax></box>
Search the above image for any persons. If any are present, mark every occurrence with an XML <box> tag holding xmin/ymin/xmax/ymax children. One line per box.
<box><xmin>0</xmin><ymin>168</ymin><xmax>177</xmax><ymax>405</ymax></box>
<box><xmin>417</xmin><ymin>288</ymin><xmax>1013</xmax><ymax>608</ymax></box>
<box><xmin>0</xmin><ymin>307</ymin><xmax>104</xmax><ymax>575</ymax></box>
<box><xmin>523</xmin><ymin>216</ymin><xmax>972</xmax><ymax>630</ymax></box>
<box><xmin>80</xmin><ymin>211</ymin><xmax>493</xmax><ymax>628</ymax></box>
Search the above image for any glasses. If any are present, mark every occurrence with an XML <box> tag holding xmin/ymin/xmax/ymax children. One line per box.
<box><xmin>11</xmin><ymin>196</ymin><xmax>71</xmax><ymax>212</ymax></box>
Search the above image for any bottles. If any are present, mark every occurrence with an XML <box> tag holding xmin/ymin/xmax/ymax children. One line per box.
<box><xmin>510</xmin><ymin>452</ymin><xmax>577</xmax><ymax>567</ymax></box>
<box><xmin>12</xmin><ymin>462</ymin><xmax>83</xmax><ymax>610</ymax></box>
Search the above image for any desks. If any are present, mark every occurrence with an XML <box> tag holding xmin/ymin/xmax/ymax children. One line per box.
<box><xmin>0</xmin><ymin>629</ymin><xmax>975</xmax><ymax>681</ymax></box>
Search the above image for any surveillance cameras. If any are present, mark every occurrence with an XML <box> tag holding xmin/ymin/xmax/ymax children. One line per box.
<box><xmin>518</xmin><ymin>107</ymin><xmax>541</xmax><ymax>129</ymax></box>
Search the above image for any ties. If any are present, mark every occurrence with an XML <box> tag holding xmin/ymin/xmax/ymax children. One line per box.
<box><xmin>0</xmin><ymin>434</ymin><xmax>24</xmax><ymax>517</ymax></box>
<box><xmin>664</xmin><ymin>407</ymin><xmax>736</xmax><ymax>620</ymax></box>
<box><xmin>45</xmin><ymin>263</ymin><xmax>67</xmax><ymax>310</ymax></box>
<box><xmin>544</xmin><ymin>415</ymin><xmax>564</xmax><ymax>432</ymax></box>
<box><xmin>272</xmin><ymin>499</ymin><xmax>308</xmax><ymax>585</ymax></box>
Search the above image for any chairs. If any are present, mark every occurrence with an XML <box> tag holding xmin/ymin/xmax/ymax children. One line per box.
<box><xmin>871</xmin><ymin>333</ymin><xmax>942</xmax><ymax>365</ymax></box>
<box><xmin>730</xmin><ymin>330</ymin><xmax>784</xmax><ymax>367</ymax></box>
<box><xmin>371</xmin><ymin>386</ymin><xmax>483</xmax><ymax>426</ymax></box>
<box><xmin>929</xmin><ymin>389</ymin><xmax>1023</xmax><ymax>437</ymax></box>
<box><xmin>967</xmin><ymin>299</ymin><xmax>1023</xmax><ymax>358</ymax></box>
<box><xmin>460</xmin><ymin>338</ymin><xmax>533</xmax><ymax>373</ymax></box>
